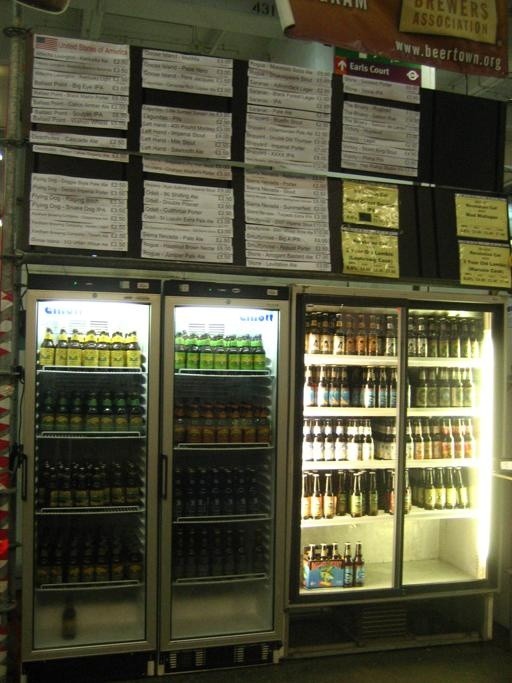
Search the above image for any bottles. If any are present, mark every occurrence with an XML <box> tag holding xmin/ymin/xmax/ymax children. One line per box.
<box><xmin>42</xmin><ymin>328</ymin><xmax>159</xmax><ymax>640</ymax></box>
<box><xmin>404</xmin><ymin>312</ymin><xmax>482</xmax><ymax>518</ymax></box>
<box><xmin>174</xmin><ymin>329</ymin><xmax>271</xmax><ymax>584</ymax></box>
<box><xmin>297</xmin><ymin>310</ymin><xmax>402</xmax><ymax>595</ymax></box>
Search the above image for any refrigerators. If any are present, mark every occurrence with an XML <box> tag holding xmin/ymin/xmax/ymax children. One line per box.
<box><xmin>20</xmin><ymin>273</ymin><xmax>289</xmax><ymax>683</ymax></box>
<box><xmin>293</xmin><ymin>296</ymin><xmax>501</xmax><ymax>658</ymax></box>
